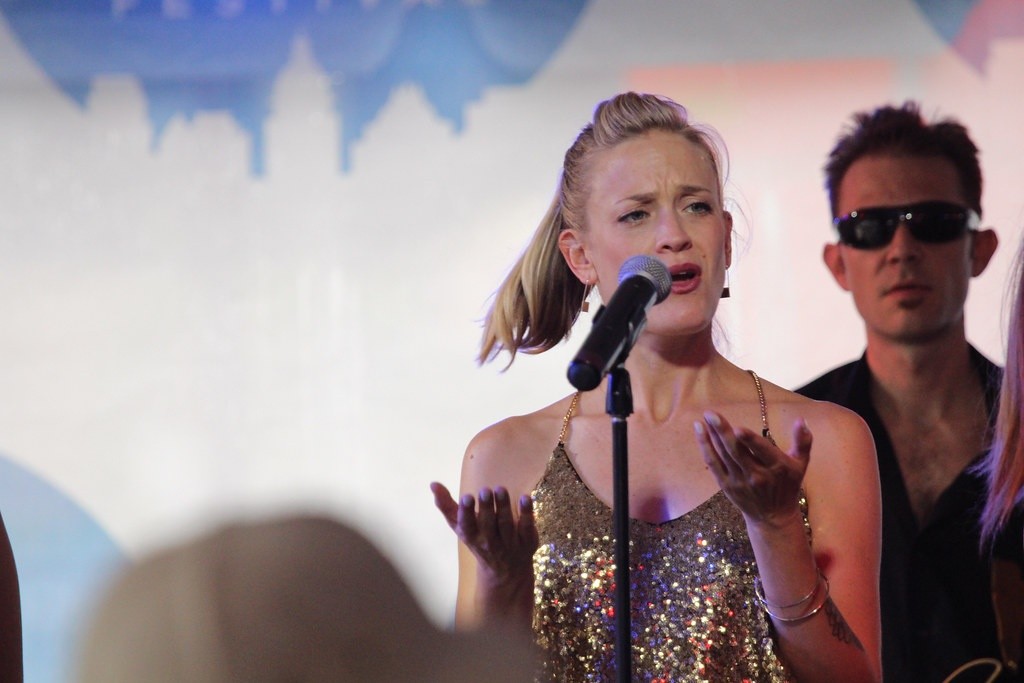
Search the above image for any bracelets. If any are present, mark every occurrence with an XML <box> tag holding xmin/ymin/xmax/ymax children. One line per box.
<box><xmin>760</xmin><ymin>572</ymin><xmax>831</xmax><ymax>621</ymax></box>
<box><xmin>754</xmin><ymin>564</ymin><xmax>819</xmax><ymax>606</ymax></box>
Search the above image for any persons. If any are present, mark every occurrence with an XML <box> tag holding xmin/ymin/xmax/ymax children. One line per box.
<box><xmin>429</xmin><ymin>90</ymin><xmax>884</xmax><ymax>683</ymax></box>
<box><xmin>0</xmin><ymin>510</ymin><xmax>547</xmax><ymax>683</ymax></box>
<box><xmin>780</xmin><ymin>106</ymin><xmax>1024</xmax><ymax>683</ymax></box>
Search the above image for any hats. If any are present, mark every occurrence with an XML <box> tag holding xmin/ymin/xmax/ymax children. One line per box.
<box><xmin>84</xmin><ymin>513</ymin><xmax>533</xmax><ymax>683</ymax></box>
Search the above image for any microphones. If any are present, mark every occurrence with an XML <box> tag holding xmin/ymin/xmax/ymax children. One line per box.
<box><xmin>567</xmin><ymin>256</ymin><xmax>672</xmax><ymax>389</ymax></box>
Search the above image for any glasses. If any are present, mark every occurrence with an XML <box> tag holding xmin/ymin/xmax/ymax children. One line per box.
<box><xmin>837</xmin><ymin>201</ymin><xmax>981</xmax><ymax>248</ymax></box>
